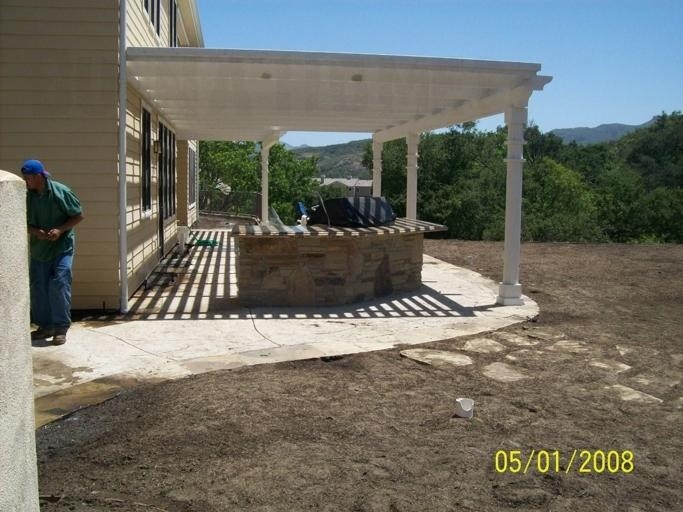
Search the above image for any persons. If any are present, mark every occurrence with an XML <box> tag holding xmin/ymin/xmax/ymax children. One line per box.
<box><xmin>21</xmin><ymin>160</ymin><xmax>85</xmax><ymax>344</ymax></box>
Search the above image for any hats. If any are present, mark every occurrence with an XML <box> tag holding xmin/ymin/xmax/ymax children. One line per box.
<box><xmin>20</xmin><ymin>160</ymin><xmax>53</xmax><ymax>179</ymax></box>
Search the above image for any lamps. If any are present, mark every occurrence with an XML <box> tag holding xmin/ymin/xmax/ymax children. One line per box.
<box><xmin>151</xmin><ymin>140</ymin><xmax>161</xmax><ymax>154</ymax></box>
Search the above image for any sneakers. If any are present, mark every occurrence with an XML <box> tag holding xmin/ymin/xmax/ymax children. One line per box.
<box><xmin>52</xmin><ymin>328</ymin><xmax>67</xmax><ymax>345</ymax></box>
<box><xmin>31</xmin><ymin>326</ymin><xmax>56</xmax><ymax>340</ymax></box>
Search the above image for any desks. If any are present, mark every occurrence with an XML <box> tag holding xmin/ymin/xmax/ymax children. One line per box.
<box><xmin>229</xmin><ymin>215</ymin><xmax>450</xmax><ymax>308</ymax></box>
